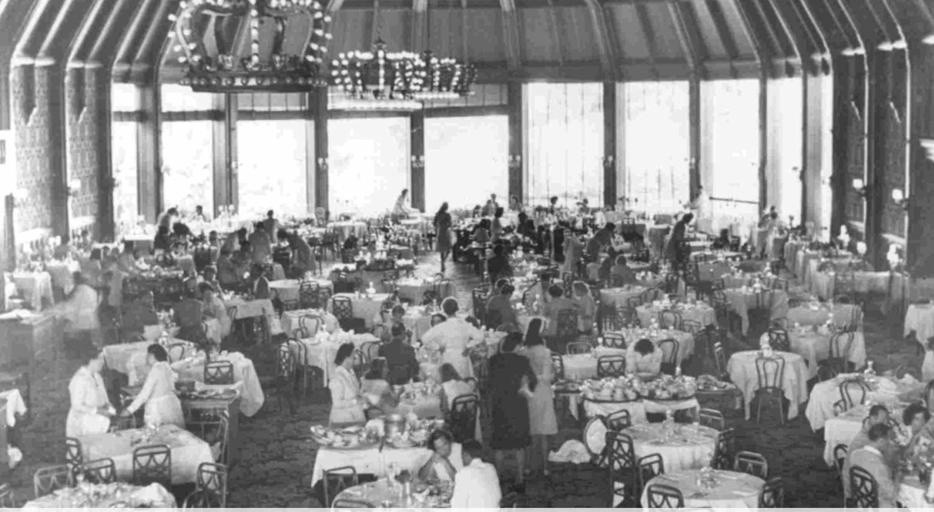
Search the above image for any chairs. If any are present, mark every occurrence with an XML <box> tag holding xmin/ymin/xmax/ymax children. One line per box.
<box><xmin>2</xmin><ymin>177</ymin><xmax>932</xmax><ymax>510</ymax></box>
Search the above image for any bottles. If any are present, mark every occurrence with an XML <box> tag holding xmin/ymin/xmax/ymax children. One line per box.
<box><xmin>863</xmin><ymin>359</ymin><xmax>876</xmax><ymax>381</ymax></box>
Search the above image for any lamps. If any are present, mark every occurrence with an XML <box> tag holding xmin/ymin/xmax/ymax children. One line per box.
<box><xmin>405</xmin><ymin>52</ymin><xmax>474</xmax><ymax>101</ymax></box>
<box><xmin>174</xmin><ymin>0</ymin><xmax>331</xmax><ymax>93</ymax></box>
<box><xmin>332</xmin><ymin>43</ymin><xmax>426</xmax><ymax>108</ymax></box>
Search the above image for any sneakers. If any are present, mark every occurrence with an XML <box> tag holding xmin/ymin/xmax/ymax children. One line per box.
<box><xmin>511</xmin><ymin>466</ymin><xmax>552</xmax><ymax>492</ymax></box>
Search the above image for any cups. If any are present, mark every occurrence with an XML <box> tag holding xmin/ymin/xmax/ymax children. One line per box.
<box><xmin>321</xmin><ymin>375</ymin><xmax>450</xmax><ymax>508</ymax></box>
<box><xmin>579</xmin><ymin>370</ymin><xmax>727</xmax><ymax>493</ymax></box>
<box><xmin>52</xmin><ymin>480</ymin><xmax>136</xmax><ymax>508</ymax></box>
